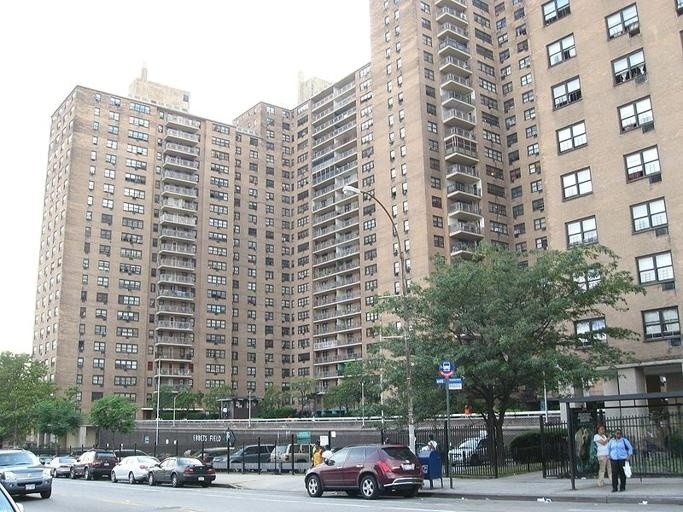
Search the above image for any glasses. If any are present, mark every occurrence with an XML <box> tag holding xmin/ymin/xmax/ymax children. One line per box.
<box><xmin>616</xmin><ymin>432</ymin><xmax>621</xmax><ymax>435</ymax></box>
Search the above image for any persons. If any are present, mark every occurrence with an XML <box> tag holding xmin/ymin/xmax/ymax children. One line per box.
<box><xmin>426</xmin><ymin>434</ymin><xmax>439</xmax><ymax>453</ymax></box>
<box><xmin>321</xmin><ymin>444</ymin><xmax>332</xmax><ymax>463</ymax></box>
<box><xmin>592</xmin><ymin>424</ymin><xmax>611</xmax><ymax>487</ymax></box>
<box><xmin>463</xmin><ymin>401</ymin><xmax>474</xmax><ymax>427</ymax></box>
<box><xmin>313</xmin><ymin>446</ymin><xmax>324</xmax><ymax>466</ymax></box>
<box><xmin>606</xmin><ymin>429</ymin><xmax>633</xmax><ymax>492</ymax></box>
<box><xmin>311</xmin><ymin>440</ymin><xmax>320</xmax><ymax>466</ymax></box>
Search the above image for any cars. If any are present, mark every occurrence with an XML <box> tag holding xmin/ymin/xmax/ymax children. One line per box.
<box><xmin>0</xmin><ymin>449</ymin><xmax>216</xmax><ymax>512</ymax></box>
<box><xmin>448</xmin><ymin>437</ymin><xmax>487</xmax><ymax>465</ymax></box>
<box><xmin>304</xmin><ymin>444</ymin><xmax>424</xmax><ymax>499</ymax></box>
<box><xmin>192</xmin><ymin>444</ymin><xmax>313</xmax><ymax>472</ymax></box>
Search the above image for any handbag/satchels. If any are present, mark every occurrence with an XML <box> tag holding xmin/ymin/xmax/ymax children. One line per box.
<box><xmin>622</xmin><ymin>460</ymin><xmax>632</xmax><ymax>477</ymax></box>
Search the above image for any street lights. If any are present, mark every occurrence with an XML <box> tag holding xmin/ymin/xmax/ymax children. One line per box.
<box><xmin>172</xmin><ymin>392</ymin><xmax>182</xmax><ymax>426</ymax></box>
<box><xmin>155</xmin><ymin>354</ymin><xmax>166</xmax><ymax>445</ymax></box>
<box><xmin>342</xmin><ymin>186</ymin><xmax>416</xmax><ymax>456</ymax></box>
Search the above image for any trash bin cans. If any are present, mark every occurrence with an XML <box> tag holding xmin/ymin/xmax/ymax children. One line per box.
<box><xmin>418</xmin><ymin>451</ymin><xmax>443</xmax><ymax>489</ymax></box>
